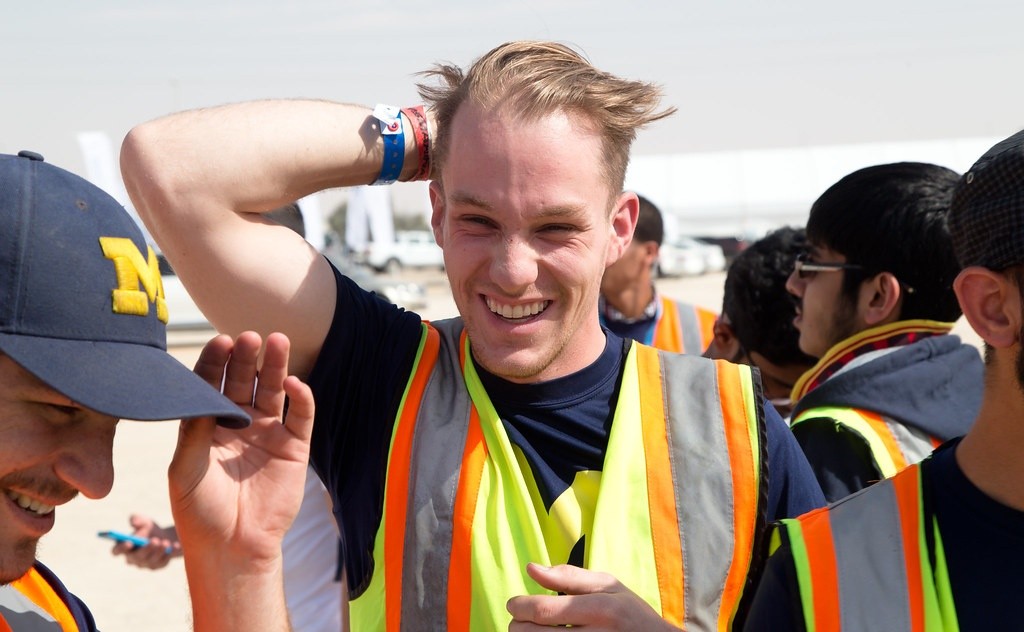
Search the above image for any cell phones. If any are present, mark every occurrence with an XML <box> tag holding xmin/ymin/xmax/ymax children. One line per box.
<box><xmin>98</xmin><ymin>529</ymin><xmax>174</xmax><ymax>558</ymax></box>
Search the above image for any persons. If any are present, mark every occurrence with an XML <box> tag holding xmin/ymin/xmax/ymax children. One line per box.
<box><xmin>600</xmin><ymin>194</ymin><xmax>719</xmax><ymax>355</ymax></box>
<box><xmin>0</xmin><ymin>150</ymin><xmax>316</xmax><ymax>632</ymax></box>
<box><xmin>118</xmin><ymin>42</ymin><xmax>826</xmax><ymax>632</ymax></box>
<box><xmin>743</xmin><ymin>128</ymin><xmax>1024</xmax><ymax>632</ymax></box>
<box><xmin>110</xmin><ymin>203</ymin><xmax>349</xmax><ymax>632</ymax></box>
<box><xmin>786</xmin><ymin>162</ymin><xmax>985</xmax><ymax>501</ymax></box>
<box><xmin>697</xmin><ymin>228</ymin><xmax>811</xmax><ymax>417</ymax></box>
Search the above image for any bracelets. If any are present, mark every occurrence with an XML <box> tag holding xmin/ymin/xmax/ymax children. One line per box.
<box><xmin>371</xmin><ymin>103</ymin><xmax>433</xmax><ymax>185</ymax></box>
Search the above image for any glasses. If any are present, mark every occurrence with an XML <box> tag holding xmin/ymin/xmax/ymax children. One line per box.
<box><xmin>713</xmin><ymin>319</ymin><xmax>794</xmax><ymax>420</ymax></box>
<box><xmin>794</xmin><ymin>249</ymin><xmax>914</xmax><ymax>294</ymax></box>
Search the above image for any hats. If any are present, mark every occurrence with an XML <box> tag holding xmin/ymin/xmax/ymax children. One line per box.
<box><xmin>0</xmin><ymin>151</ymin><xmax>252</xmax><ymax>430</ymax></box>
<box><xmin>946</xmin><ymin>129</ymin><xmax>1024</xmax><ymax>274</ymax></box>
